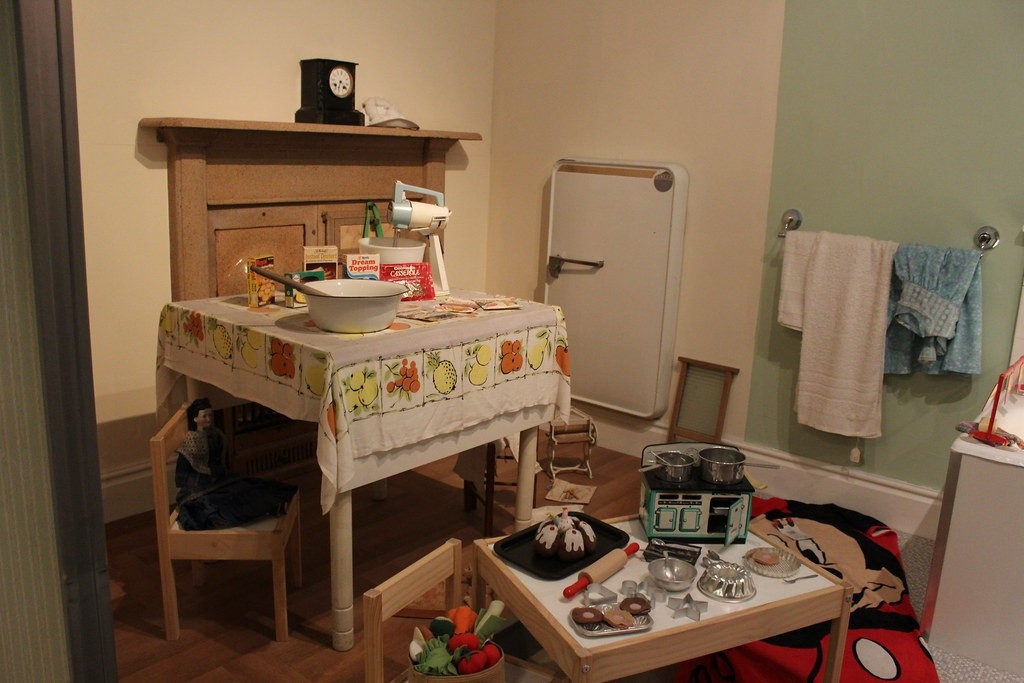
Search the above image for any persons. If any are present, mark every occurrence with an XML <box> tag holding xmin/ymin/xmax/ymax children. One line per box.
<box><xmin>172</xmin><ymin>396</ymin><xmax>291</xmax><ymax>532</ymax></box>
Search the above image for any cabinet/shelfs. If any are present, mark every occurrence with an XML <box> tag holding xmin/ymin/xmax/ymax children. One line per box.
<box><xmin>205</xmin><ymin>199</ymin><xmax>423</xmax><ymax>436</ymax></box>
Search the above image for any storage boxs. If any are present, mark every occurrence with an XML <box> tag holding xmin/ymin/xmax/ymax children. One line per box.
<box><xmin>246</xmin><ymin>245</ymin><xmax>436</xmax><ymax>308</ymax></box>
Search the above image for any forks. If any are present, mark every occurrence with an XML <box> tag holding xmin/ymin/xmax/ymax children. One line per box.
<box><xmin>784</xmin><ymin>575</ymin><xmax>819</xmax><ymax>584</ymax></box>
<box><xmin>639</xmin><ymin>548</ymin><xmax>693</xmax><ymax>562</ymax></box>
<box><xmin>708</xmin><ymin>550</ymin><xmax>728</xmax><ymax>563</ymax></box>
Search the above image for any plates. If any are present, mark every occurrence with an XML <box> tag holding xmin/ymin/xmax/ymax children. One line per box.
<box><xmin>494</xmin><ymin>511</ymin><xmax>630</xmax><ymax>580</ymax></box>
<box><xmin>570</xmin><ymin>603</ymin><xmax>654</xmax><ymax>637</ymax></box>
<box><xmin>744</xmin><ymin>548</ymin><xmax>801</xmax><ymax>578</ymax></box>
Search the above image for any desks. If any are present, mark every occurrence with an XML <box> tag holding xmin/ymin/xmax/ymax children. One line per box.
<box><xmin>472</xmin><ymin>514</ymin><xmax>853</xmax><ymax>683</ymax></box>
<box><xmin>154</xmin><ymin>285</ymin><xmax>572</xmax><ymax>653</ymax></box>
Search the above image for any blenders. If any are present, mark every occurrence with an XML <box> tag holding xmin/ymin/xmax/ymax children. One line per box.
<box><xmin>387</xmin><ymin>178</ymin><xmax>452</xmax><ymax>297</ymax></box>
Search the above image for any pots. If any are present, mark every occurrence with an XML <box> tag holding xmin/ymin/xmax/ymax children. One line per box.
<box><xmin>697</xmin><ymin>448</ymin><xmax>781</xmax><ymax>485</ymax></box>
<box><xmin>637</xmin><ymin>452</ymin><xmax>694</xmax><ymax>483</ymax></box>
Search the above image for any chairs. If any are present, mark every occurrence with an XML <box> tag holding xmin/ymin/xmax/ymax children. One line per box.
<box><xmin>362</xmin><ymin>538</ymin><xmax>569</xmax><ymax>683</ymax></box>
<box><xmin>149</xmin><ymin>402</ymin><xmax>302</xmax><ymax>644</ymax></box>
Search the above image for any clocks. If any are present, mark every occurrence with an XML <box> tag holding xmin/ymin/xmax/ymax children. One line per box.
<box><xmin>329</xmin><ymin>66</ymin><xmax>353</xmax><ymax>98</ymax></box>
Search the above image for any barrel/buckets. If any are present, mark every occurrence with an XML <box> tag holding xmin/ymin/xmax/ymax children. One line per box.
<box><xmin>357</xmin><ymin>237</ymin><xmax>427</xmax><ymax>264</ymax></box>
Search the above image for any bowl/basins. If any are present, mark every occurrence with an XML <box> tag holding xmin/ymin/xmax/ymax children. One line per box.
<box><xmin>698</xmin><ymin>562</ymin><xmax>758</xmax><ymax>603</ymax></box>
<box><xmin>647</xmin><ymin>557</ymin><xmax>698</xmax><ymax>591</ymax></box>
<box><xmin>303</xmin><ymin>279</ymin><xmax>409</xmax><ymax>333</ymax></box>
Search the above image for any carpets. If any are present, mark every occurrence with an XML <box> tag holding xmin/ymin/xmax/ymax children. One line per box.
<box><xmin>671</xmin><ymin>496</ymin><xmax>941</xmax><ymax>682</ymax></box>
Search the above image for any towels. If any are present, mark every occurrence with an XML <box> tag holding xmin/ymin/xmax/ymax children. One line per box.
<box><xmin>777</xmin><ymin>230</ymin><xmax>817</xmax><ymax>334</ymax></box>
<box><xmin>789</xmin><ymin>231</ymin><xmax>899</xmax><ymax>438</ymax></box>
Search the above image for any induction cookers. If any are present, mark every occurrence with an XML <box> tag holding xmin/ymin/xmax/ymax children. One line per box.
<box><xmin>638</xmin><ymin>441</ymin><xmax>756</xmax><ymax>547</ymax></box>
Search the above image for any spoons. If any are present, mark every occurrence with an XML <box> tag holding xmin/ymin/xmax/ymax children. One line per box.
<box><xmin>702</xmin><ymin>557</ymin><xmax>712</xmax><ymax>567</ymax></box>
<box><xmin>651</xmin><ymin>538</ymin><xmax>700</xmax><ymax>552</ymax></box>
